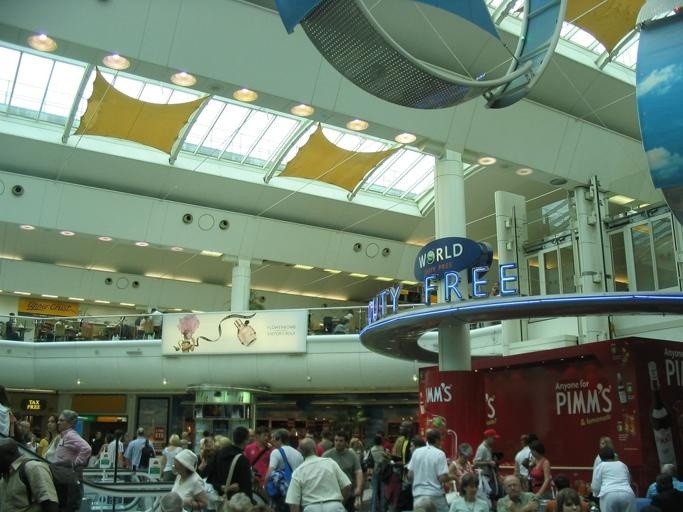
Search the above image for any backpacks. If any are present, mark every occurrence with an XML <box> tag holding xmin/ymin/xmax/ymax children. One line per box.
<box><xmin>140</xmin><ymin>439</ymin><xmax>154</xmax><ymax>466</ymax></box>
<box><xmin>20</xmin><ymin>458</ymin><xmax>83</xmax><ymax>512</ymax></box>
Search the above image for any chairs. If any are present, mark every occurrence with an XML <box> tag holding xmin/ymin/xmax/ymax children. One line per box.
<box><xmin>306</xmin><ymin>308</ymin><xmax>356</xmax><ymax>335</ymax></box>
<box><xmin>0</xmin><ymin>310</ymin><xmax>171</xmax><ymax>342</ymax></box>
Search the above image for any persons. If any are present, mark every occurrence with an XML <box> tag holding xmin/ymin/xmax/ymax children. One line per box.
<box><xmin>6</xmin><ymin>308</ymin><xmax>162</xmax><ymax>342</ymax></box>
<box><xmin>320</xmin><ymin>303</ymin><xmax>355</xmax><ymax>334</ymax></box>
<box><xmin>483</xmin><ymin>282</ymin><xmax>500</xmax><ymax>327</ymax></box>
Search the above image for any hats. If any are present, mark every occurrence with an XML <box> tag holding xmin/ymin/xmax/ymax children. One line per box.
<box><xmin>483</xmin><ymin>429</ymin><xmax>500</xmax><ymax>439</ymax></box>
<box><xmin>175</xmin><ymin>448</ymin><xmax>198</xmax><ymax>473</ymax></box>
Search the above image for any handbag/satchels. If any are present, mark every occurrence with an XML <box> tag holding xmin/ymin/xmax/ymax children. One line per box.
<box><xmin>220</xmin><ymin>496</ymin><xmax>235</xmax><ymax>512</ymax></box>
<box><xmin>481</xmin><ymin>474</ymin><xmax>505</xmax><ymax>500</ymax></box>
<box><xmin>384</xmin><ymin>472</ymin><xmax>403</xmax><ymax>508</ymax></box>
<box><xmin>521</xmin><ymin>458</ymin><xmax>531</xmax><ymax>468</ymax></box>
<box><xmin>266</xmin><ymin>467</ymin><xmax>292</xmax><ymax>497</ymax></box>
<box><xmin>445</xmin><ymin>491</ymin><xmax>460</xmax><ymax>506</ymax></box>
<box><xmin>367</xmin><ymin>453</ymin><xmax>374</xmax><ymax>468</ymax></box>
